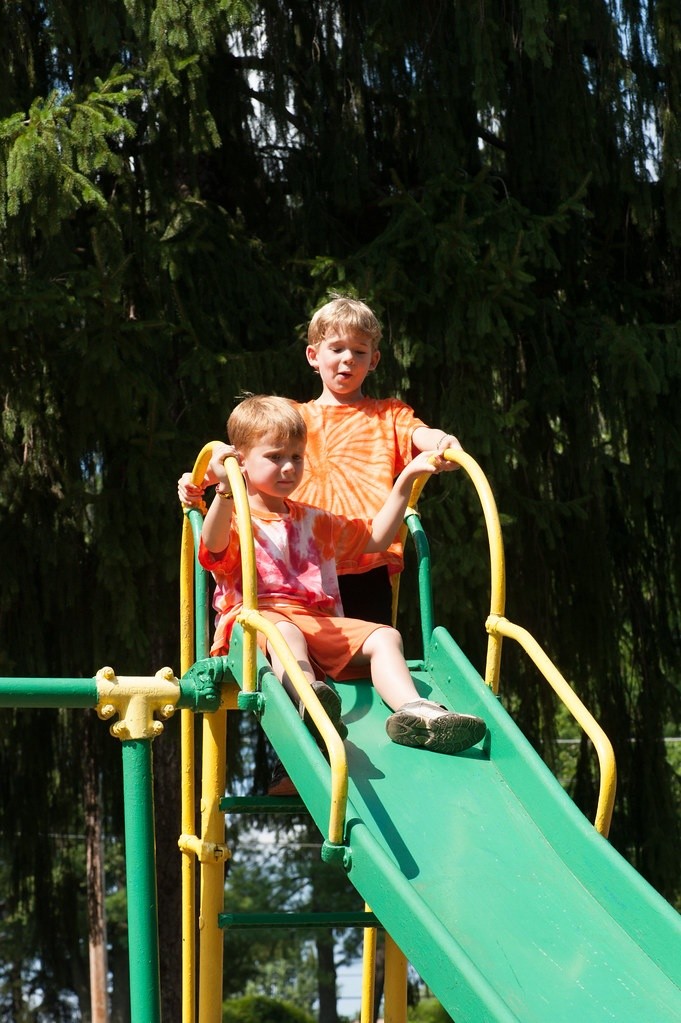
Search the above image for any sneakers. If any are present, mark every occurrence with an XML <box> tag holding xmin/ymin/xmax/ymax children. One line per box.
<box><xmin>299</xmin><ymin>680</ymin><xmax>349</xmax><ymax>749</ymax></box>
<box><xmin>385</xmin><ymin>698</ymin><xmax>487</xmax><ymax>755</ymax></box>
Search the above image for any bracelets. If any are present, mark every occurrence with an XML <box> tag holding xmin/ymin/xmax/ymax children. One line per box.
<box><xmin>215</xmin><ymin>484</ymin><xmax>233</xmax><ymax>499</ymax></box>
<box><xmin>437</xmin><ymin>435</ymin><xmax>447</xmax><ymax>450</ymax></box>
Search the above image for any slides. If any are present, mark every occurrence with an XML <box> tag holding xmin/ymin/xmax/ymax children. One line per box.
<box><xmin>216</xmin><ymin>566</ymin><xmax>681</xmax><ymax>1022</ymax></box>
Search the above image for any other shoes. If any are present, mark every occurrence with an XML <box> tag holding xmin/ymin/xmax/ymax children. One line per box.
<box><xmin>268</xmin><ymin>776</ymin><xmax>299</xmax><ymax>797</ymax></box>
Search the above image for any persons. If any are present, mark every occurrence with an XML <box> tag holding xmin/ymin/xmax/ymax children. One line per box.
<box><xmin>178</xmin><ymin>297</ymin><xmax>464</xmax><ymax>795</ymax></box>
<box><xmin>199</xmin><ymin>396</ymin><xmax>486</xmax><ymax>754</ymax></box>
